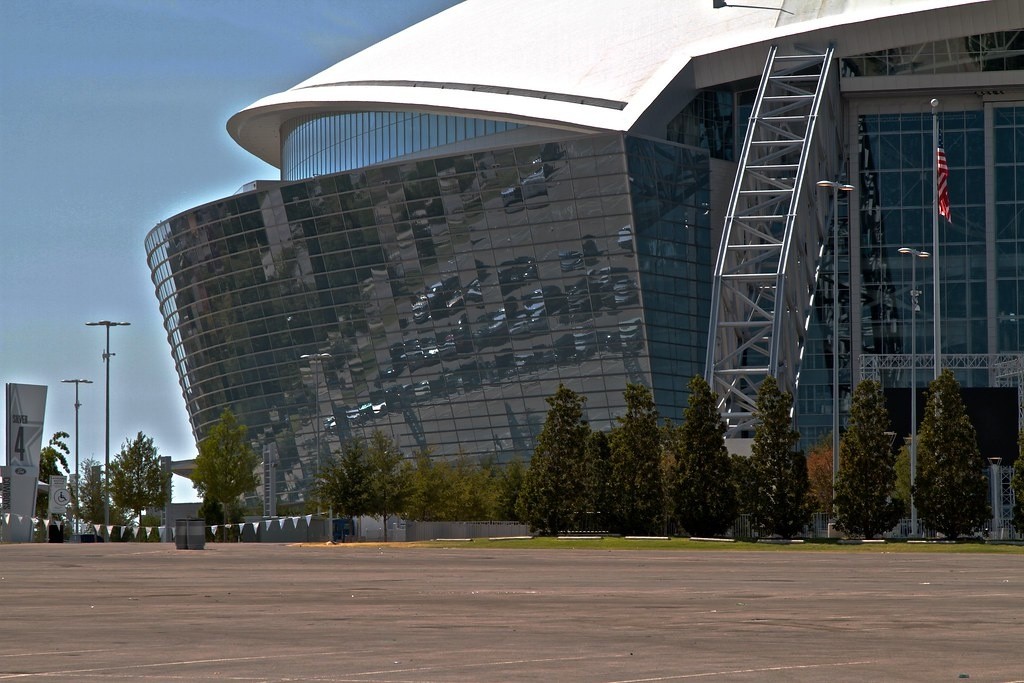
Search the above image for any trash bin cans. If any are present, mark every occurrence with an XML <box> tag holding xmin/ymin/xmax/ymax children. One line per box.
<box><xmin>175</xmin><ymin>519</ymin><xmax>205</xmax><ymax>550</ymax></box>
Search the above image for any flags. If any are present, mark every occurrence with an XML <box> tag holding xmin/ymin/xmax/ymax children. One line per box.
<box><xmin>937</xmin><ymin>118</ymin><xmax>952</xmax><ymax>225</ymax></box>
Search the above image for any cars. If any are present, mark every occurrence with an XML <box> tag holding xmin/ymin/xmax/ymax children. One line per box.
<box><xmin>320</xmin><ymin>177</ymin><xmax>686</xmax><ymax>435</ymax></box>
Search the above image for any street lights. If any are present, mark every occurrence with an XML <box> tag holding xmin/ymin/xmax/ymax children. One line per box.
<box><xmin>898</xmin><ymin>247</ymin><xmax>931</xmax><ymax>543</ymax></box>
<box><xmin>816</xmin><ymin>180</ymin><xmax>855</xmax><ymax>544</ymax></box>
<box><xmin>85</xmin><ymin>320</ymin><xmax>131</xmax><ymax>525</ymax></box>
<box><xmin>62</xmin><ymin>378</ymin><xmax>93</xmax><ymax>542</ymax></box>
<box><xmin>300</xmin><ymin>352</ymin><xmax>332</xmax><ymax>516</ymax></box>
